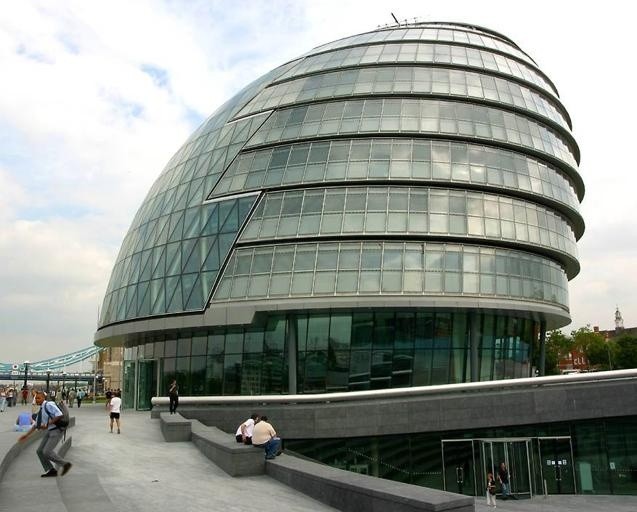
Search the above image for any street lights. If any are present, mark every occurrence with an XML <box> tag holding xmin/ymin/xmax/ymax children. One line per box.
<box><xmin>102</xmin><ymin>378</ymin><xmax>108</xmax><ymax>393</ymax></box>
<box><xmin>23</xmin><ymin>361</ymin><xmax>31</xmax><ymax>405</ymax></box>
<box><xmin>46</xmin><ymin>368</ymin><xmax>51</xmax><ymax>391</ymax></box>
<box><xmin>62</xmin><ymin>372</ymin><xmax>67</xmax><ymax>389</ymax></box>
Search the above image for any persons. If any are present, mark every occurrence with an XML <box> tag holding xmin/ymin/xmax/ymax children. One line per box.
<box><xmin>167</xmin><ymin>379</ymin><xmax>179</xmax><ymax>416</ymax></box>
<box><xmin>107</xmin><ymin>393</ymin><xmax>121</xmax><ymax>434</ymax></box>
<box><xmin>0</xmin><ymin>383</ymin><xmax>121</xmax><ymax>412</ymax></box>
<box><xmin>235</xmin><ymin>413</ymin><xmax>283</xmax><ymax>460</ymax></box>
<box><xmin>18</xmin><ymin>388</ymin><xmax>72</xmax><ymax>477</ymax></box>
<box><xmin>485</xmin><ymin>463</ymin><xmax>509</xmax><ymax>510</ymax></box>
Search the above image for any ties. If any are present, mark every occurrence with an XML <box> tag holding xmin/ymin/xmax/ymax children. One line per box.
<box><xmin>37</xmin><ymin>407</ymin><xmax>42</xmax><ymax>431</ymax></box>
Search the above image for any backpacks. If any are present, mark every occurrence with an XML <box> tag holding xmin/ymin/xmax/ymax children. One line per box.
<box><xmin>44</xmin><ymin>401</ymin><xmax>69</xmax><ymax>427</ymax></box>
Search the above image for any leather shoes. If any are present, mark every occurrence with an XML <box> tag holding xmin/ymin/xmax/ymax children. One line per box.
<box><xmin>61</xmin><ymin>462</ymin><xmax>72</xmax><ymax>476</ymax></box>
<box><xmin>41</xmin><ymin>470</ymin><xmax>57</xmax><ymax>477</ymax></box>
